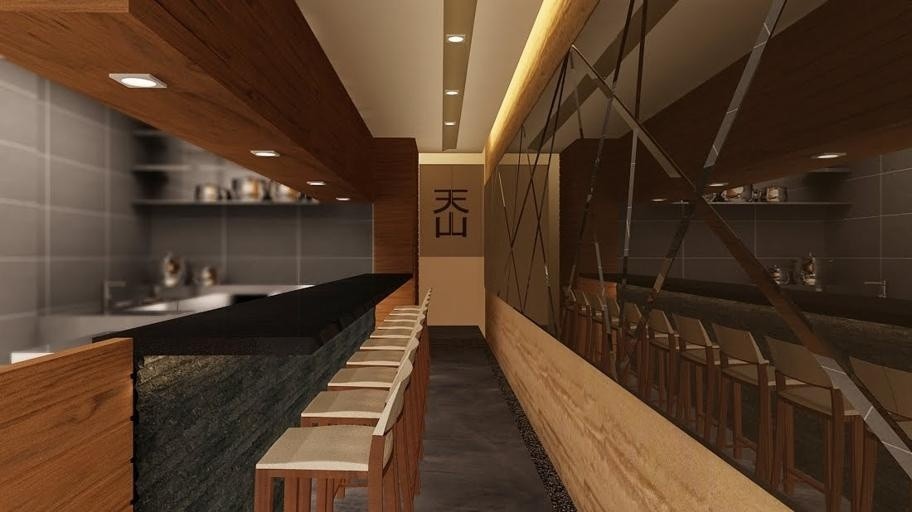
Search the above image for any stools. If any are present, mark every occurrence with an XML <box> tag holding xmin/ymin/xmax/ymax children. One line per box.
<box><xmin>562</xmin><ymin>286</ymin><xmax>912</xmax><ymax>509</ymax></box>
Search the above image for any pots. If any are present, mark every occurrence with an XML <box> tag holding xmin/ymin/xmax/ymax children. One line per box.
<box><xmin>194</xmin><ymin>174</ymin><xmax>317</xmax><ymax>203</ymax></box>
<box><xmin>701</xmin><ymin>184</ymin><xmax>788</xmax><ymax>204</ymax></box>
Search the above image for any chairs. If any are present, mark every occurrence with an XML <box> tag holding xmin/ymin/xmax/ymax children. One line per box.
<box><xmin>255</xmin><ymin>286</ymin><xmax>432</xmax><ymax>510</ymax></box>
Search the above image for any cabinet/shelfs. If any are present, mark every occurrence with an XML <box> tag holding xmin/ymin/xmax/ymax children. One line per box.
<box><xmin>129</xmin><ymin>128</ymin><xmax>322</xmax><ymax>207</ymax></box>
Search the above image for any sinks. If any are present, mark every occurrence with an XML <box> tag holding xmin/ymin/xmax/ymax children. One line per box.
<box><xmin>95</xmin><ymin>291</ymin><xmax>282</xmax><ymax>317</ymax></box>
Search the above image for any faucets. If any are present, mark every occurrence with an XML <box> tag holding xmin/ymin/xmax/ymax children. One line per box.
<box><xmin>863</xmin><ymin>278</ymin><xmax>888</xmax><ymax>299</ymax></box>
<box><xmin>101</xmin><ymin>280</ymin><xmax>125</xmax><ymax>307</ymax></box>
